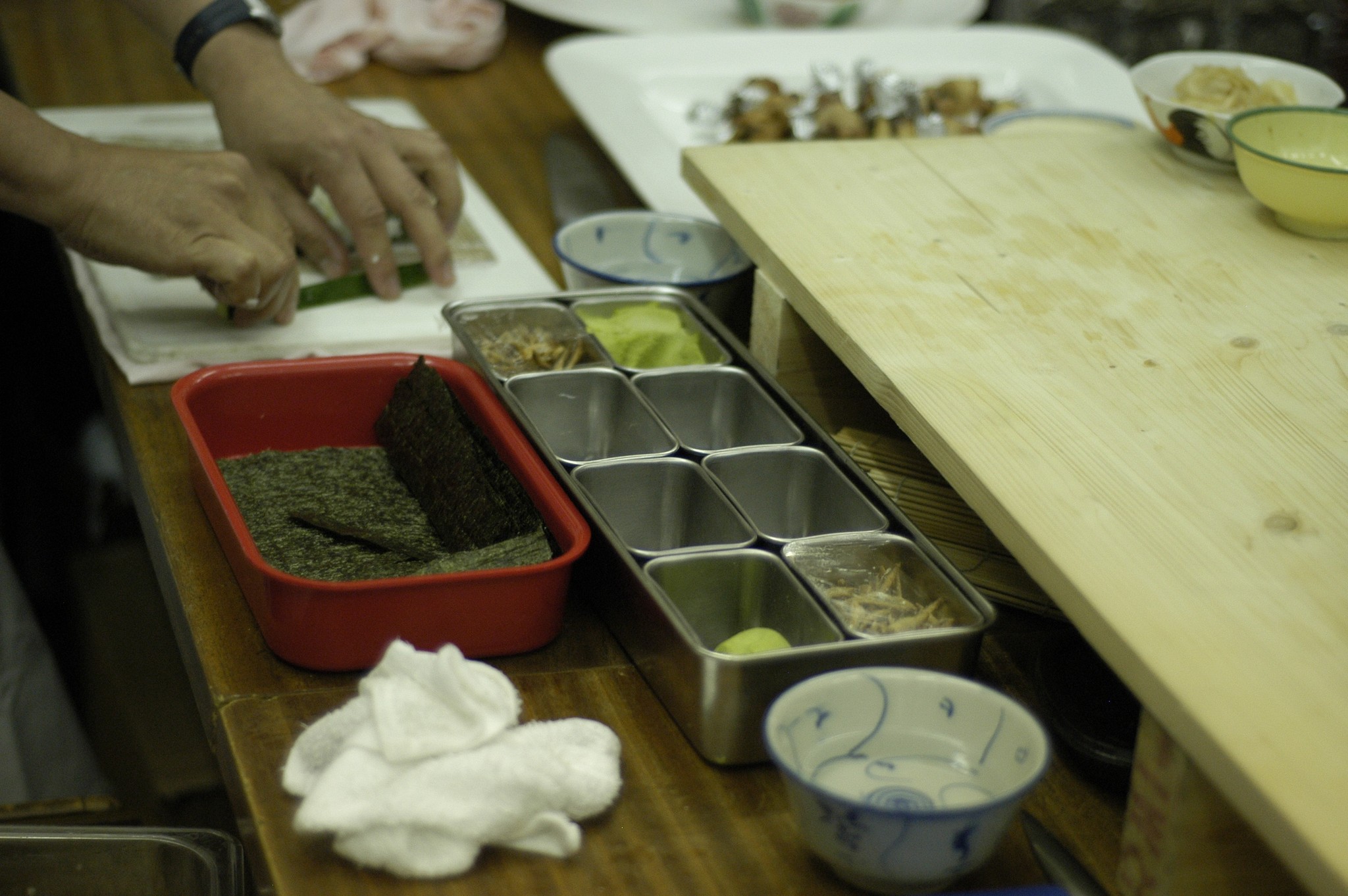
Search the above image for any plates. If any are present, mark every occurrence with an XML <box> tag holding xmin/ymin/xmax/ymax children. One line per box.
<box><xmin>511</xmin><ymin>0</ymin><xmax>987</xmax><ymax>33</ymax></box>
<box><xmin>543</xmin><ymin>25</ymin><xmax>1154</xmax><ymax>217</ymax></box>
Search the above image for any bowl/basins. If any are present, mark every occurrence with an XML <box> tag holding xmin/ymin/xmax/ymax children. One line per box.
<box><xmin>171</xmin><ymin>354</ymin><xmax>588</xmax><ymax>670</ymax></box>
<box><xmin>763</xmin><ymin>665</ymin><xmax>1050</xmax><ymax>896</ymax></box>
<box><xmin>1130</xmin><ymin>50</ymin><xmax>1343</xmax><ymax>173</ymax></box>
<box><xmin>552</xmin><ymin>209</ymin><xmax>753</xmax><ymax>321</ymax></box>
<box><xmin>454</xmin><ymin>294</ymin><xmax>984</xmax><ymax>656</ymax></box>
<box><xmin>1224</xmin><ymin>104</ymin><xmax>1348</xmax><ymax>242</ymax></box>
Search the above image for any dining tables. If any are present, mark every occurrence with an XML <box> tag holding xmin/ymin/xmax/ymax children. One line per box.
<box><xmin>0</xmin><ymin>0</ymin><xmax>1348</xmax><ymax>895</ymax></box>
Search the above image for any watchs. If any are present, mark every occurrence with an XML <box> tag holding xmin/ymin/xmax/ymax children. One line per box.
<box><xmin>174</xmin><ymin>0</ymin><xmax>284</xmax><ymax>84</ymax></box>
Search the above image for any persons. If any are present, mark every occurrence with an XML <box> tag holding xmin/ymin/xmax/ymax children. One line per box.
<box><xmin>0</xmin><ymin>0</ymin><xmax>463</xmax><ymax>795</ymax></box>
